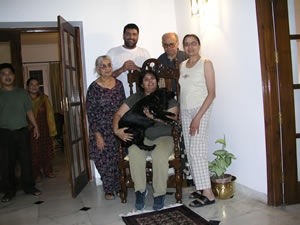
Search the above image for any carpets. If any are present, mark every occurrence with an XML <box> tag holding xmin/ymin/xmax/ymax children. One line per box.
<box><xmin>120</xmin><ymin>203</ymin><xmax>216</xmax><ymax>225</ymax></box>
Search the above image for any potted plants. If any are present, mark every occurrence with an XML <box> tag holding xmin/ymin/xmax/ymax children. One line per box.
<box><xmin>208</xmin><ymin>135</ymin><xmax>236</xmax><ymax>200</ymax></box>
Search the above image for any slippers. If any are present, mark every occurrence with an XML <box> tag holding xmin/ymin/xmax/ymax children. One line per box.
<box><xmin>189</xmin><ymin>190</ymin><xmax>204</xmax><ymax>199</ymax></box>
<box><xmin>24</xmin><ymin>187</ymin><xmax>41</xmax><ymax>195</ymax></box>
<box><xmin>45</xmin><ymin>172</ymin><xmax>56</xmax><ymax>178</ymax></box>
<box><xmin>33</xmin><ymin>172</ymin><xmax>43</xmax><ymax>183</ymax></box>
<box><xmin>1</xmin><ymin>190</ymin><xmax>17</xmax><ymax>202</ymax></box>
<box><xmin>189</xmin><ymin>195</ymin><xmax>216</xmax><ymax>207</ymax></box>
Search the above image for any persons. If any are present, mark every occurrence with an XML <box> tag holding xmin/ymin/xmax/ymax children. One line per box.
<box><xmin>0</xmin><ymin>63</ymin><xmax>40</xmax><ymax>202</ymax></box>
<box><xmin>106</xmin><ymin>23</ymin><xmax>155</xmax><ymax>99</ymax></box>
<box><xmin>25</xmin><ymin>76</ymin><xmax>57</xmax><ymax>183</ymax></box>
<box><xmin>153</xmin><ymin>32</ymin><xmax>189</xmax><ymax>93</ymax></box>
<box><xmin>86</xmin><ymin>55</ymin><xmax>126</xmax><ymax>200</ymax></box>
<box><xmin>177</xmin><ymin>34</ymin><xmax>215</xmax><ymax>208</ymax></box>
<box><xmin>112</xmin><ymin>70</ymin><xmax>178</xmax><ymax>211</ymax></box>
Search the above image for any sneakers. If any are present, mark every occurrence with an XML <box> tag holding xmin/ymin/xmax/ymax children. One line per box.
<box><xmin>152</xmin><ymin>196</ymin><xmax>165</xmax><ymax>210</ymax></box>
<box><xmin>134</xmin><ymin>188</ymin><xmax>147</xmax><ymax>210</ymax></box>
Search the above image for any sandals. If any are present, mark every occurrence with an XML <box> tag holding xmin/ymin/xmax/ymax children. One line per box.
<box><xmin>119</xmin><ymin>192</ymin><xmax>127</xmax><ymax>203</ymax></box>
<box><xmin>104</xmin><ymin>190</ymin><xmax>116</xmax><ymax>200</ymax></box>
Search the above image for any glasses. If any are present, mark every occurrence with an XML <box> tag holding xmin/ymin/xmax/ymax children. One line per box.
<box><xmin>163</xmin><ymin>40</ymin><xmax>178</xmax><ymax>49</ymax></box>
<box><xmin>99</xmin><ymin>63</ymin><xmax>112</xmax><ymax>69</ymax></box>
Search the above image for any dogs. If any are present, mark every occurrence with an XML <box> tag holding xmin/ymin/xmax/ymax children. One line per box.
<box><xmin>118</xmin><ymin>87</ymin><xmax>176</xmax><ymax>157</ymax></box>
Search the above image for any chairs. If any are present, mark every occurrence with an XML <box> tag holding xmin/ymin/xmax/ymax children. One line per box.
<box><xmin>118</xmin><ymin>58</ymin><xmax>185</xmax><ymax>203</ymax></box>
